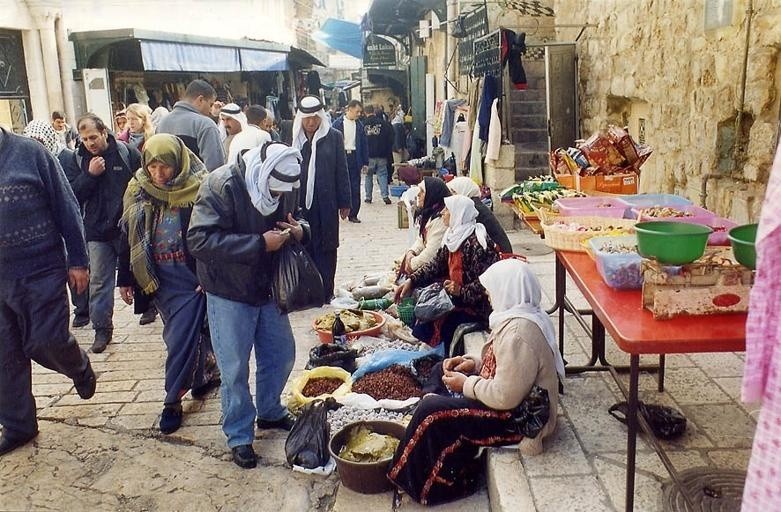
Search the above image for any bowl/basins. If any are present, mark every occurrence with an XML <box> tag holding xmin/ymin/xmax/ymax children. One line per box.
<box><xmin>728</xmin><ymin>223</ymin><xmax>760</xmax><ymax>271</ymax></box>
<box><xmin>329</xmin><ymin>419</ymin><xmax>409</xmax><ymax>495</ymax></box>
<box><xmin>634</xmin><ymin>220</ymin><xmax>713</xmax><ymax>267</ymax></box>
<box><xmin>313</xmin><ymin>309</ymin><xmax>385</xmax><ymax>345</ymax></box>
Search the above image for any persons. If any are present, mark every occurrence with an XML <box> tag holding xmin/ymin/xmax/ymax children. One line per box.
<box><xmin>325</xmin><ymin>99</ymin><xmax>406</xmax><ymax>224</ymax></box>
<box><xmin>0</xmin><ymin>125</ymin><xmax>95</xmax><ymax>455</ymax></box>
<box><xmin>394</xmin><ymin>176</ymin><xmax>512</xmax><ymax>357</ymax></box>
<box><xmin>382</xmin><ymin>258</ymin><xmax>565</xmax><ymax>507</ymax></box>
<box><xmin>115</xmin><ymin>131</ymin><xmax>221</xmax><ymax>435</ymax></box>
<box><xmin>183</xmin><ymin>140</ymin><xmax>310</xmax><ymax>468</ymax></box>
<box><xmin>23</xmin><ymin>78</ymin><xmax>280</xmax><ymax>354</ymax></box>
<box><xmin>279</xmin><ymin>95</ymin><xmax>353</xmax><ymax>306</ymax></box>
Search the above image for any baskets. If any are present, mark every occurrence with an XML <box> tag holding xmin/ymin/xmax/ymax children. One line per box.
<box><xmin>396</xmin><ymin>297</ymin><xmax>417</xmax><ymax>328</ymax></box>
<box><xmin>539</xmin><ymin>206</ymin><xmax>643</xmax><ymax>254</ymax></box>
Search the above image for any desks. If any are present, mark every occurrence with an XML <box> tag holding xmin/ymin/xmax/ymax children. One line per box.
<box><xmin>499</xmin><ymin>188</ymin><xmax>657</xmax><ymax>374</ymax></box>
<box><xmin>556</xmin><ymin>245</ymin><xmax>747</xmax><ymax>512</ymax></box>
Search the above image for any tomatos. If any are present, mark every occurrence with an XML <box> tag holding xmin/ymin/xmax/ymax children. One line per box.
<box><xmin>713</xmin><ymin>294</ymin><xmax>740</xmax><ymax>307</ymax></box>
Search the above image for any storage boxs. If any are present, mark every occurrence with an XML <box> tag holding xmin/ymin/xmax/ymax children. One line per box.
<box><xmin>553</xmin><ymin>172</ymin><xmax>741</xmax><ymax>289</ymax></box>
<box><xmin>397</xmin><ymin>197</ymin><xmax>408</xmax><ymax>229</ymax></box>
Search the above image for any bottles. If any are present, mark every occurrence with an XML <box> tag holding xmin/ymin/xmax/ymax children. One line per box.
<box><xmin>332</xmin><ymin>313</ymin><xmax>346</xmax><ymax>344</ymax></box>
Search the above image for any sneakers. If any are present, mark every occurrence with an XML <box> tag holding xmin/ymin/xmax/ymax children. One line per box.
<box><xmin>139</xmin><ymin>305</ymin><xmax>158</xmax><ymax>325</ymax></box>
<box><xmin>159</xmin><ymin>400</ymin><xmax>183</xmax><ymax>435</ymax></box>
<box><xmin>92</xmin><ymin>327</ymin><xmax>113</xmax><ymax>353</ymax></box>
<box><xmin>232</xmin><ymin>443</ymin><xmax>257</xmax><ymax>469</ymax></box>
<box><xmin>256</xmin><ymin>411</ymin><xmax>297</xmax><ymax>431</ymax></box>
<box><xmin>364</xmin><ymin>198</ymin><xmax>372</xmax><ymax>203</ymax></box>
<box><xmin>191</xmin><ymin>377</ymin><xmax>222</xmax><ymax>400</ymax></box>
<box><xmin>383</xmin><ymin>197</ymin><xmax>391</xmax><ymax>205</ymax></box>
<box><xmin>73</xmin><ymin>313</ymin><xmax>90</xmax><ymax>327</ymax></box>
<box><xmin>0</xmin><ymin>423</ymin><xmax>40</xmax><ymax>457</ymax></box>
<box><xmin>347</xmin><ymin>215</ymin><xmax>361</xmax><ymax>223</ymax></box>
<box><xmin>72</xmin><ymin>357</ymin><xmax>96</xmax><ymax>399</ymax></box>
